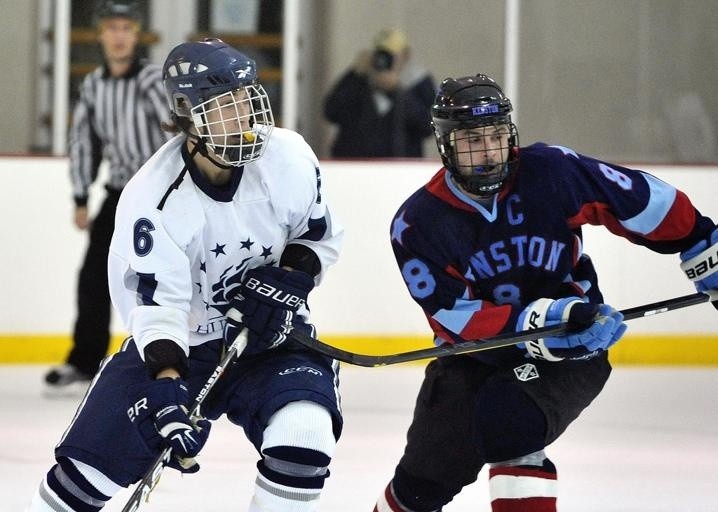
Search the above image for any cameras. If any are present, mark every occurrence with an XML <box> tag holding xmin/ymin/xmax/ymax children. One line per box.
<box><xmin>370</xmin><ymin>51</ymin><xmax>391</xmax><ymax>71</ymax></box>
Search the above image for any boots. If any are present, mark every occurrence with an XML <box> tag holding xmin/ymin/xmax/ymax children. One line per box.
<box><xmin>44</xmin><ymin>365</ymin><xmax>94</xmax><ymax>384</ymax></box>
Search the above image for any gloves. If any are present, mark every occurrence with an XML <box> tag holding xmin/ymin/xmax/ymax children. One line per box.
<box><xmin>514</xmin><ymin>296</ymin><xmax>627</xmax><ymax>362</ymax></box>
<box><xmin>127</xmin><ymin>377</ymin><xmax>211</xmax><ymax>473</ymax></box>
<box><xmin>226</xmin><ymin>266</ymin><xmax>314</xmax><ymax>356</ymax></box>
<box><xmin>680</xmin><ymin>227</ymin><xmax>718</xmax><ymax>310</ymax></box>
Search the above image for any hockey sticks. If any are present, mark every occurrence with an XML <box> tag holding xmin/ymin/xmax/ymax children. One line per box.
<box><xmin>288</xmin><ymin>292</ymin><xmax>713</xmax><ymax>368</ymax></box>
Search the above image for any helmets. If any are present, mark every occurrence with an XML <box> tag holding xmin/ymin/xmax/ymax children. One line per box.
<box><xmin>95</xmin><ymin>2</ymin><xmax>142</xmax><ymax>21</ymax></box>
<box><xmin>164</xmin><ymin>38</ymin><xmax>257</xmax><ymax>119</ymax></box>
<box><xmin>431</xmin><ymin>74</ymin><xmax>515</xmax><ymax>193</ymax></box>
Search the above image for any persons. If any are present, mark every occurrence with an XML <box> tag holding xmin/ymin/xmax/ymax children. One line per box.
<box><xmin>42</xmin><ymin>4</ymin><xmax>176</xmax><ymax>386</ymax></box>
<box><xmin>321</xmin><ymin>30</ymin><xmax>436</xmax><ymax>156</ymax></box>
<box><xmin>39</xmin><ymin>35</ymin><xmax>344</xmax><ymax>512</ymax></box>
<box><xmin>372</xmin><ymin>68</ymin><xmax>716</xmax><ymax>512</ymax></box>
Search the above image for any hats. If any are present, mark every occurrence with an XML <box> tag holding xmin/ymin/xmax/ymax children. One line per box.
<box><xmin>375</xmin><ymin>27</ymin><xmax>406</xmax><ymax>54</ymax></box>
